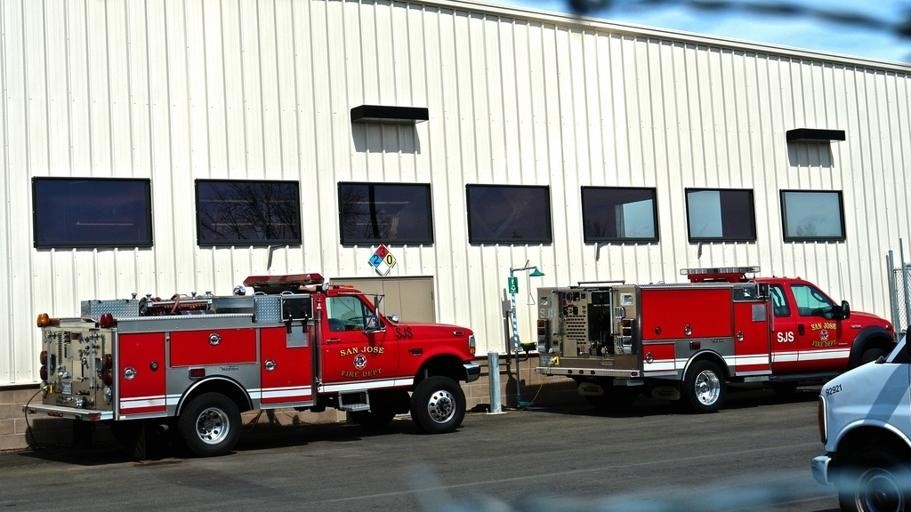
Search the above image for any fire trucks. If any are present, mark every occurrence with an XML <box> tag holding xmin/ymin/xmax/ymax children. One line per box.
<box><xmin>533</xmin><ymin>265</ymin><xmax>894</xmax><ymax>413</ymax></box>
<box><xmin>20</xmin><ymin>273</ymin><xmax>482</xmax><ymax>454</ymax></box>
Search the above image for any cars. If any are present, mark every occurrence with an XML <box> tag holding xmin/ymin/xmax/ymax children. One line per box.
<box><xmin>811</xmin><ymin>324</ymin><xmax>911</xmax><ymax>512</ymax></box>
